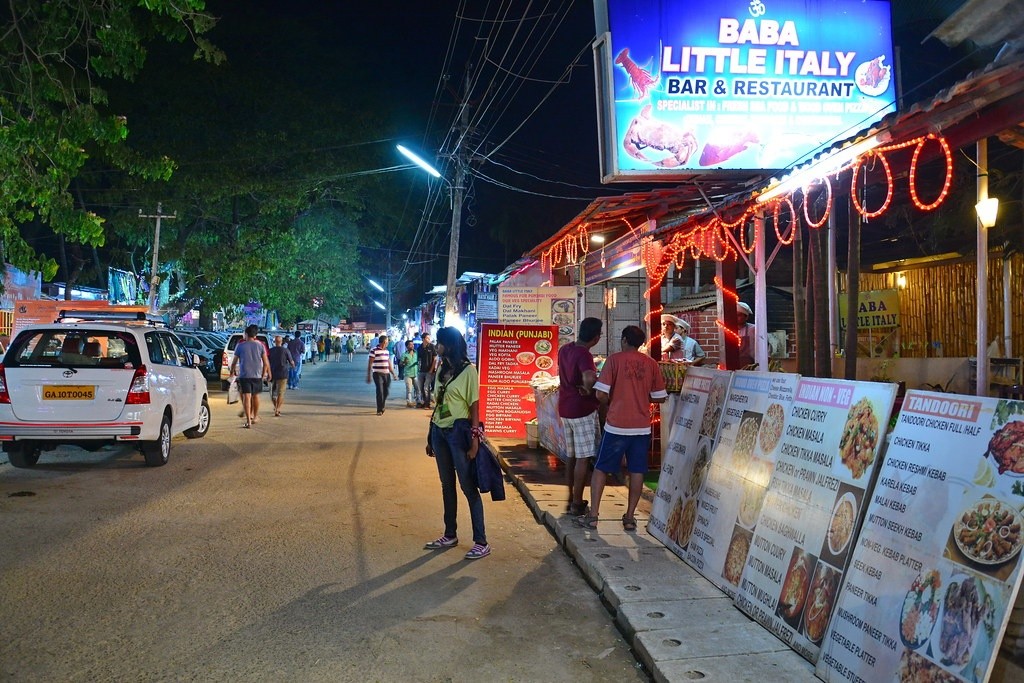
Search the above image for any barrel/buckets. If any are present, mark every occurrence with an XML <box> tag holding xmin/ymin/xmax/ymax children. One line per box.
<box><xmin>523</xmin><ymin>421</ymin><xmax>539</xmax><ymax>449</ymax></box>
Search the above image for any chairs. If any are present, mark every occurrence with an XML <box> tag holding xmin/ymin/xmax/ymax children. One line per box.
<box><xmin>62</xmin><ymin>338</ymin><xmax>101</xmax><ymax>358</ymax></box>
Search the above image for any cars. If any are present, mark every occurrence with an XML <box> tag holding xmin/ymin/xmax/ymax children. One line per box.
<box><xmin>174</xmin><ymin>329</ymin><xmax>227</xmax><ymax>374</ymax></box>
<box><xmin>272</xmin><ymin>330</ymin><xmax>319</xmax><ymax>365</ymax></box>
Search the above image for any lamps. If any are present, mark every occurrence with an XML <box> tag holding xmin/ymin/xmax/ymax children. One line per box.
<box><xmin>755</xmin><ymin>130</ymin><xmax>894</xmax><ymax>203</ymax></box>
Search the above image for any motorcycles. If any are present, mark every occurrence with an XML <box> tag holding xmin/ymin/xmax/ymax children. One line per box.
<box><xmin>206</xmin><ymin>348</ymin><xmax>224</xmax><ymax>377</ymax></box>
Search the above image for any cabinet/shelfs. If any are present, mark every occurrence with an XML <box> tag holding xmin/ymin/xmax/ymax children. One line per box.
<box><xmin>969</xmin><ymin>356</ymin><xmax>1023</xmax><ymax>400</ymax></box>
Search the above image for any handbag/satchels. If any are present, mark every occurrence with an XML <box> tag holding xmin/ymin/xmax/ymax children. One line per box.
<box><xmin>352</xmin><ymin>349</ymin><xmax>355</xmax><ymax>353</ymax></box>
<box><xmin>227</xmin><ymin>375</ymin><xmax>239</xmax><ymax>404</ymax></box>
<box><xmin>426</xmin><ymin>421</ymin><xmax>435</xmax><ymax>457</ymax></box>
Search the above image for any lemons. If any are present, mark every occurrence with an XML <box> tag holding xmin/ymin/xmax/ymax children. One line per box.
<box><xmin>972</xmin><ymin>456</ymin><xmax>996</xmax><ymax>488</ymax></box>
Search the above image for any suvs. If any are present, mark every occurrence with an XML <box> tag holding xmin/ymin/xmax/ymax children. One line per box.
<box><xmin>220</xmin><ymin>333</ymin><xmax>272</xmax><ymax>390</ymax></box>
<box><xmin>0</xmin><ymin>310</ymin><xmax>210</xmax><ymax>467</ymax></box>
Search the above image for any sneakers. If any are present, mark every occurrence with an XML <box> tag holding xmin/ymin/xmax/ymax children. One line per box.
<box><xmin>426</xmin><ymin>537</ymin><xmax>458</xmax><ymax>548</ymax></box>
<box><xmin>465</xmin><ymin>544</ymin><xmax>490</xmax><ymax>559</ymax></box>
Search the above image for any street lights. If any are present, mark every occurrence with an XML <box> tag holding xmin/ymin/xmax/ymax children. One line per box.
<box><xmin>395</xmin><ymin>141</ymin><xmax>467</xmax><ymax>331</ymax></box>
<box><xmin>369</xmin><ymin>280</ymin><xmax>392</xmax><ymax>329</ymax></box>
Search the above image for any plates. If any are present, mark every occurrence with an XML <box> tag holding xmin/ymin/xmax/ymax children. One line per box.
<box><xmin>553</xmin><ymin>299</ymin><xmax>574</xmax><ymax>346</ymax></box>
<box><xmin>531</xmin><ymin>371</ymin><xmax>551</xmax><ymax>381</ymax></box>
<box><xmin>535</xmin><ymin>356</ymin><xmax>553</xmax><ymax>369</ymax></box>
<box><xmin>989</xmin><ymin>451</ymin><xmax>1024</xmax><ymax>477</ymax></box>
<box><xmin>516</xmin><ymin>352</ymin><xmax>536</xmax><ymax>365</ymax></box>
<box><xmin>690</xmin><ymin>436</ymin><xmax>712</xmax><ymax>499</ymax></box>
<box><xmin>898</xmin><ymin>572</ymin><xmax>983</xmax><ymax>673</ymax></box>
<box><xmin>665</xmin><ymin>494</ymin><xmax>699</xmax><ymax>548</ymax></box>
<box><xmin>804</xmin><ymin>611</ymin><xmax>824</xmax><ymax>642</ymax></box>
<box><xmin>534</xmin><ymin>340</ymin><xmax>552</xmax><ymax>354</ymax></box>
<box><xmin>737</xmin><ymin>508</ymin><xmax>756</xmax><ymax>530</ymax></box>
<box><xmin>953</xmin><ymin>498</ymin><xmax>1024</xmax><ymax>564</ymax></box>
<box><xmin>827</xmin><ymin>491</ymin><xmax>859</xmax><ymax>555</ymax></box>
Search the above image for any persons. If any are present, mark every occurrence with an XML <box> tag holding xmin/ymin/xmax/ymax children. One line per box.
<box><xmin>312</xmin><ymin>334</ymin><xmax>359</xmax><ymax>364</ymax></box>
<box><xmin>425</xmin><ymin>326</ymin><xmax>491</xmax><ymax>560</ymax></box>
<box><xmin>736</xmin><ymin>301</ymin><xmax>755</xmax><ymax>368</ymax></box>
<box><xmin>674</xmin><ymin>317</ymin><xmax>705</xmax><ymax>366</ymax></box>
<box><xmin>417</xmin><ymin>333</ymin><xmax>437</xmax><ymax>409</ymax></box>
<box><xmin>400</xmin><ymin>341</ymin><xmax>422</xmax><ymax>407</ymax></box>
<box><xmin>558</xmin><ymin>317</ymin><xmax>603</xmax><ymax>514</ymax></box>
<box><xmin>369</xmin><ymin>333</ymin><xmax>438</xmax><ymax>380</ymax></box>
<box><xmin>570</xmin><ymin>325</ymin><xmax>667</xmax><ymax>531</ymax></box>
<box><xmin>367</xmin><ymin>335</ymin><xmax>397</xmax><ymax>415</ymax></box>
<box><xmin>230</xmin><ymin>324</ymin><xmax>306</xmax><ymax>428</ymax></box>
<box><xmin>660</xmin><ymin>314</ymin><xmax>683</xmax><ymax>358</ymax></box>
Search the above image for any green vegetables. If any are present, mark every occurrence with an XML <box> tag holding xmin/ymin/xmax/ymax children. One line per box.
<box><xmin>971</xmin><ymin>508</ymin><xmax>1004</xmax><ymax>542</ymax></box>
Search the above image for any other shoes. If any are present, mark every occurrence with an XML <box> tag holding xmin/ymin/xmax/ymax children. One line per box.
<box><xmin>252</xmin><ymin>418</ymin><xmax>260</xmax><ymax>424</ymax></box>
<box><xmin>289</xmin><ymin>386</ymin><xmax>299</xmax><ymax>390</ymax></box>
<box><xmin>407</xmin><ymin>402</ymin><xmax>430</xmax><ymax>410</ymax></box>
<box><xmin>377</xmin><ymin>411</ymin><xmax>384</xmax><ymax>415</ymax></box>
<box><xmin>567</xmin><ymin>500</ymin><xmax>599</xmax><ymax>515</ymax></box>
<box><xmin>243</xmin><ymin>423</ymin><xmax>250</xmax><ymax>428</ymax></box>
<box><xmin>274</xmin><ymin>409</ymin><xmax>280</xmax><ymax>416</ymax></box>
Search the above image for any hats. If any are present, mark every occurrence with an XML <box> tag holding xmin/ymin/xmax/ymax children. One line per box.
<box><xmin>661</xmin><ymin>314</ymin><xmax>691</xmax><ymax>331</ymax></box>
<box><xmin>737</xmin><ymin>302</ymin><xmax>754</xmax><ymax>315</ymax></box>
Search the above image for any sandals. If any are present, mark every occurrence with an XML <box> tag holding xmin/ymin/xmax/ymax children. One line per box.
<box><xmin>571</xmin><ymin>512</ymin><xmax>598</xmax><ymax>530</ymax></box>
<box><xmin>622</xmin><ymin>513</ymin><xmax>637</xmax><ymax>531</ymax></box>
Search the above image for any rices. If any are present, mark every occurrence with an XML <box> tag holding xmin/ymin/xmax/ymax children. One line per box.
<box><xmin>901</xmin><ymin>569</ymin><xmax>940</xmax><ymax>644</ymax></box>
